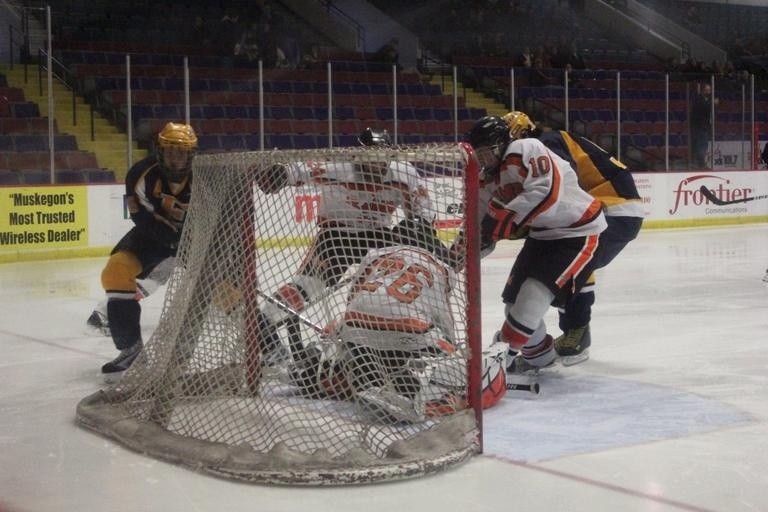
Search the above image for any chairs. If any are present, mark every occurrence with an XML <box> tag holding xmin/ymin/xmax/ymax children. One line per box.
<box><xmin>0</xmin><ymin>0</ymin><xmax>767</xmax><ymax>188</ymax></box>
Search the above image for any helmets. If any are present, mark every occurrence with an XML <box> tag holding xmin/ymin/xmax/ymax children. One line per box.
<box><xmin>354</xmin><ymin>126</ymin><xmax>394</xmax><ymax>182</ymax></box>
<box><xmin>465</xmin><ymin>111</ymin><xmax>536</xmax><ymax>176</ymax></box>
<box><xmin>155</xmin><ymin>122</ymin><xmax>200</xmax><ymax>187</ymax></box>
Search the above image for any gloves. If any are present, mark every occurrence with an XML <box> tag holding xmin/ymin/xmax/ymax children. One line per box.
<box><xmin>257</xmin><ymin>164</ymin><xmax>287</xmax><ymax>195</ymax></box>
<box><xmin>481</xmin><ymin>199</ymin><xmax>518</xmax><ymax>244</ymax></box>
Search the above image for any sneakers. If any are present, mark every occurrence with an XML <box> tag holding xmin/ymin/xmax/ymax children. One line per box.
<box><xmin>102</xmin><ymin>334</ymin><xmax>145</xmax><ymax>373</ymax></box>
<box><xmin>553</xmin><ymin>323</ymin><xmax>591</xmax><ymax>357</ymax></box>
<box><xmin>507</xmin><ymin>354</ymin><xmax>556</xmax><ymax>375</ymax></box>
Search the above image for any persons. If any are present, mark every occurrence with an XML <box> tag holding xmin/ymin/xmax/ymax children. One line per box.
<box><xmin>370</xmin><ymin>39</ymin><xmax>404</xmax><ymax>72</ymax></box>
<box><xmin>281</xmin><ymin>244</ymin><xmax>507</xmax><ymax>425</ymax></box>
<box><xmin>502</xmin><ymin>111</ymin><xmax>645</xmax><ymax>356</ymax></box>
<box><xmin>184</xmin><ymin>0</ymin><xmax>322</xmax><ymax>71</ymax></box>
<box><xmin>88</xmin><ymin>255</ymin><xmax>178</xmax><ymax>337</ymax></box>
<box><xmin>450</xmin><ymin>115</ymin><xmax>609</xmax><ymax>375</ymax></box>
<box><xmin>254</xmin><ymin>128</ymin><xmax>448</xmax><ymax>324</ymax></box>
<box><xmin>657</xmin><ymin>4</ymin><xmax>768</xmax><ymax>169</ymax></box>
<box><xmin>437</xmin><ymin>0</ymin><xmax>587</xmax><ymax>88</ymax></box>
<box><xmin>101</xmin><ymin>122</ymin><xmax>290</xmax><ymax>374</ymax></box>
